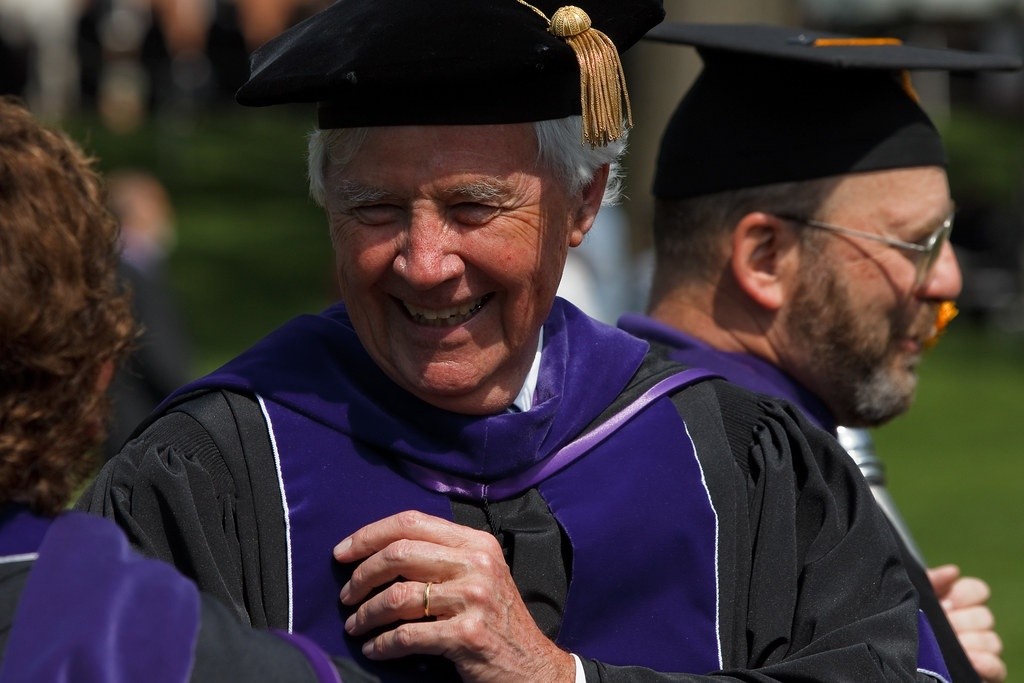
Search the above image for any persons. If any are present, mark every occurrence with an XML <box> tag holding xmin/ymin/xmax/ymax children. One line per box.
<box><xmin>74</xmin><ymin>0</ymin><xmax>946</xmax><ymax>683</ymax></box>
<box><xmin>647</xmin><ymin>21</ymin><xmax>1024</xmax><ymax>682</ymax></box>
<box><xmin>106</xmin><ymin>167</ymin><xmax>183</xmax><ymax>446</ymax></box>
<box><xmin>0</xmin><ymin>101</ymin><xmax>382</xmax><ymax>683</ymax></box>
<box><xmin>16</xmin><ymin>0</ymin><xmax>295</xmax><ymax>126</ymax></box>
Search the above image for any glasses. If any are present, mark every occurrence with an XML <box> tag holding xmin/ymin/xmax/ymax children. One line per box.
<box><xmin>772</xmin><ymin>210</ymin><xmax>954</xmax><ymax>303</ymax></box>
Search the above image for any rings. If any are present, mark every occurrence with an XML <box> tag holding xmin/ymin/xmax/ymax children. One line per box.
<box><xmin>425</xmin><ymin>581</ymin><xmax>432</xmax><ymax>618</ymax></box>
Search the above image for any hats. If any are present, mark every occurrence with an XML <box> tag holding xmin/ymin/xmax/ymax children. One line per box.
<box><xmin>231</xmin><ymin>0</ymin><xmax>668</xmax><ymax>131</ymax></box>
<box><xmin>640</xmin><ymin>21</ymin><xmax>1024</xmax><ymax>201</ymax></box>
